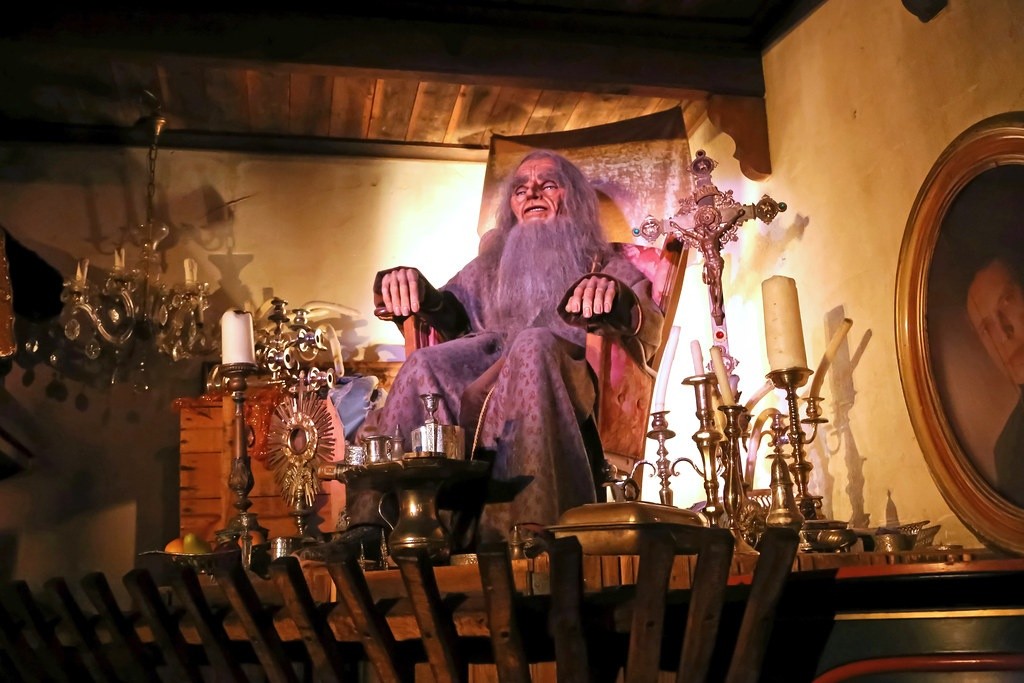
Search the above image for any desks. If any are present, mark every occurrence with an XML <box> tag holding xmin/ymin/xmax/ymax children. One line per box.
<box><xmin>132</xmin><ymin>548</ymin><xmax>1004</xmax><ymax>683</ymax></box>
<box><xmin>314</xmin><ymin>460</ymin><xmax>531</xmax><ymax>562</ymax></box>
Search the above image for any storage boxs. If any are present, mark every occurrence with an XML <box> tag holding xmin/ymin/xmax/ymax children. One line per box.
<box><xmin>174</xmin><ymin>386</ymin><xmax>408</xmax><ymax>543</ymax></box>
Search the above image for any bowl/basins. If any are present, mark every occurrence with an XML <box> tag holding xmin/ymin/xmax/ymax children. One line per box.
<box><xmin>450</xmin><ymin>553</ymin><xmax>479</xmax><ymax>566</ymax></box>
<box><xmin>798</xmin><ymin>519</ymin><xmax>848</xmax><ymax>547</ymax></box>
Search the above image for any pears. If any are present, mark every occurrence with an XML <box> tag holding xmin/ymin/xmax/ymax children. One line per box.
<box><xmin>183</xmin><ymin>534</ymin><xmax>212</xmax><ymax>553</ymax></box>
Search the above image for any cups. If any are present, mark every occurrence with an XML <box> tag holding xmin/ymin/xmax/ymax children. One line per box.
<box><xmin>345</xmin><ymin>445</ymin><xmax>365</xmax><ymax>466</ymax></box>
<box><xmin>365</xmin><ymin>435</ymin><xmax>392</xmax><ymax>466</ymax></box>
<box><xmin>875</xmin><ymin>533</ymin><xmax>906</xmax><ymax>552</ymax></box>
<box><xmin>800</xmin><ymin>526</ymin><xmax>858</xmax><ymax>553</ymax></box>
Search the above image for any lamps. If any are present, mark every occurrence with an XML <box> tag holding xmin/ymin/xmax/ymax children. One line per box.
<box><xmin>17</xmin><ymin>114</ymin><xmax>220</xmax><ymax>395</ymax></box>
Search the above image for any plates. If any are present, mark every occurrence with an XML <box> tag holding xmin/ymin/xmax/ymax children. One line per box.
<box><xmin>138</xmin><ymin>532</ymin><xmax>239</xmax><ymax>576</ymax></box>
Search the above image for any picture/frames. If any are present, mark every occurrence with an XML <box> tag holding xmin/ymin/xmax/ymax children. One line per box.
<box><xmin>893</xmin><ymin>111</ymin><xmax>1024</xmax><ymax>556</ymax></box>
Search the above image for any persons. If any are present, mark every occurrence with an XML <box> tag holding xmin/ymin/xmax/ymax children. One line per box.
<box><xmin>962</xmin><ymin>245</ymin><xmax>1024</xmax><ymax>509</ymax></box>
<box><xmin>297</xmin><ymin>153</ymin><xmax>665</xmax><ymax>560</ymax></box>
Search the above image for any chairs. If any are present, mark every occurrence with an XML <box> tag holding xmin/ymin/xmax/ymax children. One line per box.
<box><xmin>371</xmin><ymin>231</ymin><xmax>688</xmax><ymax>501</ymax></box>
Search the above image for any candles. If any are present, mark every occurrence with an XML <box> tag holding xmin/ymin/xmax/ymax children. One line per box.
<box><xmin>654</xmin><ymin>326</ymin><xmax>681</xmax><ymax>412</ymax></box>
<box><xmin>808</xmin><ymin>318</ymin><xmax>854</xmax><ymax>398</ymax></box>
<box><xmin>222</xmin><ymin>308</ymin><xmax>257</xmax><ymax>366</ymax></box>
<box><xmin>744</xmin><ymin>408</ymin><xmax>780</xmax><ymax>492</ymax></box>
<box><xmin>760</xmin><ymin>273</ymin><xmax>813</xmax><ymax>368</ymax></box>
<box><xmin>691</xmin><ymin>338</ymin><xmax>705</xmax><ymax>376</ymax></box>
<box><xmin>710</xmin><ymin>344</ymin><xmax>733</xmax><ymax>405</ymax></box>
<box><xmin>737</xmin><ymin>380</ymin><xmax>773</xmax><ymax>433</ymax></box>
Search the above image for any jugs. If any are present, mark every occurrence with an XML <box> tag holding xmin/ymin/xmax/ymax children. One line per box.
<box><xmin>378</xmin><ymin>477</ymin><xmax>452</xmax><ymax>568</ymax></box>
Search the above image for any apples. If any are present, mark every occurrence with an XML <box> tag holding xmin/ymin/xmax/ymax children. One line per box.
<box><xmin>165</xmin><ymin>538</ymin><xmax>183</xmax><ymax>553</ymax></box>
